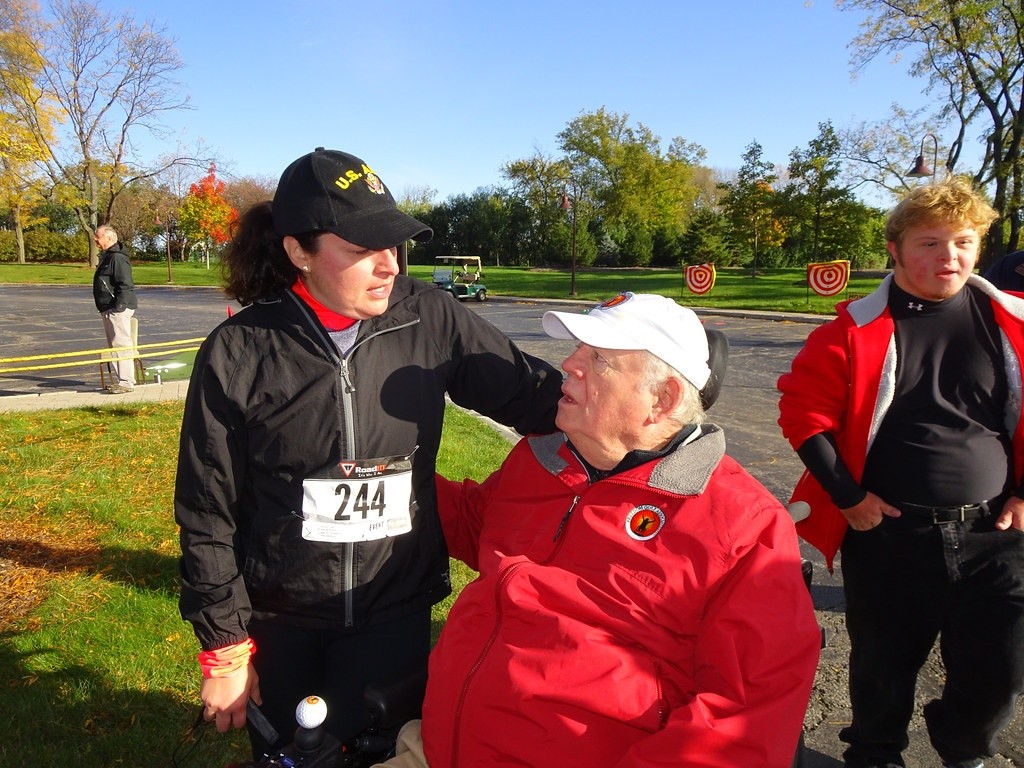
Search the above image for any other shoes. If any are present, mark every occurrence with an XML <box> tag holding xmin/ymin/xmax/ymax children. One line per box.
<box><xmin>949</xmin><ymin>752</ymin><xmax>1016</xmax><ymax>768</ymax></box>
<box><xmin>108</xmin><ymin>383</ymin><xmax>138</xmax><ymax>395</ymax></box>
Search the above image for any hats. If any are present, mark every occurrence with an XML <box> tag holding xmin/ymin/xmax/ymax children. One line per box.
<box><xmin>541</xmin><ymin>292</ymin><xmax>712</xmax><ymax>388</ymax></box>
<box><xmin>269</xmin><ymin>148</ymin><xmax>436</xmax><ymax>250</ymax></box>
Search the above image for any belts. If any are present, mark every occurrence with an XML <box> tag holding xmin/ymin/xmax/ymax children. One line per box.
<box><xmin>881</xmin><ymin>500</ymin><xmax>1002</xmax><ymax>524</ymax></box>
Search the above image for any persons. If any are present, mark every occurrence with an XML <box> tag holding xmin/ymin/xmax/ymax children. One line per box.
<box><xmin>371</xmin><ymin>290</ymin><xmax>822</xmax><ymax>768</ymax></box>
<box><xmin>777</xmin><ymin>174</ymin><xmax>1024</xmax><ymax>768</ymax></box>
<box><xmin>92</xmin><ymin>225</ymin><xmax>138</xmax><ymax>395</ymax></box>
<box><xmin>174</xmin><ymin>147</ymin><xmax>565</xmax><ymax>768</ymax></box>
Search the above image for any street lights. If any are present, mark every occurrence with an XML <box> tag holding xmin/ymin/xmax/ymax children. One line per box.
<box><xmin>152</xmin><ymin>206</ymin><xmax>173</xmax><ymax>282</ymax></box>
<box><xmin>559</xmin><ymin>182</ymin><xmax>577</xmax><ymax>295</ymax></box>
<box><xmin>906</xmin><ymin>133</ymin><xmax>937</xmax><ymax>187</ymax></box>
